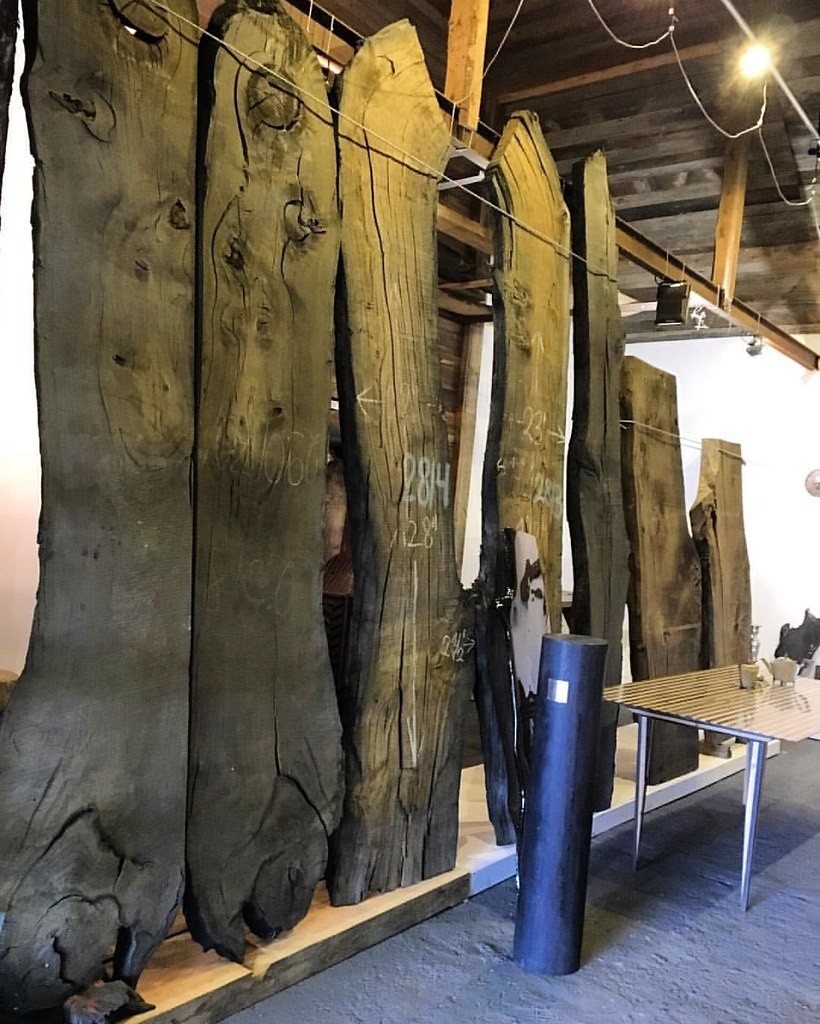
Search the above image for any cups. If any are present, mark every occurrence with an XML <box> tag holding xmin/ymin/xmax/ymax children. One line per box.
<box><xmin>739</xmin><ymin>664</ymin><xmax>759</xmax><ymax>688</ymax></box>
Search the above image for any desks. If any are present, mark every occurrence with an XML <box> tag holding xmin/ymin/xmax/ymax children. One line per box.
<box><xmin>602</xmin><ymin>664</ymin><xmax>820</xmax><ymax>913</ymax></box>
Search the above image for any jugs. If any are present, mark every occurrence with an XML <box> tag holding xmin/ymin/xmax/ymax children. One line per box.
<box><xmin>761</xmin><ymin>654</ymin><xmax>801</xmax><ymax>685</ymax></box>
